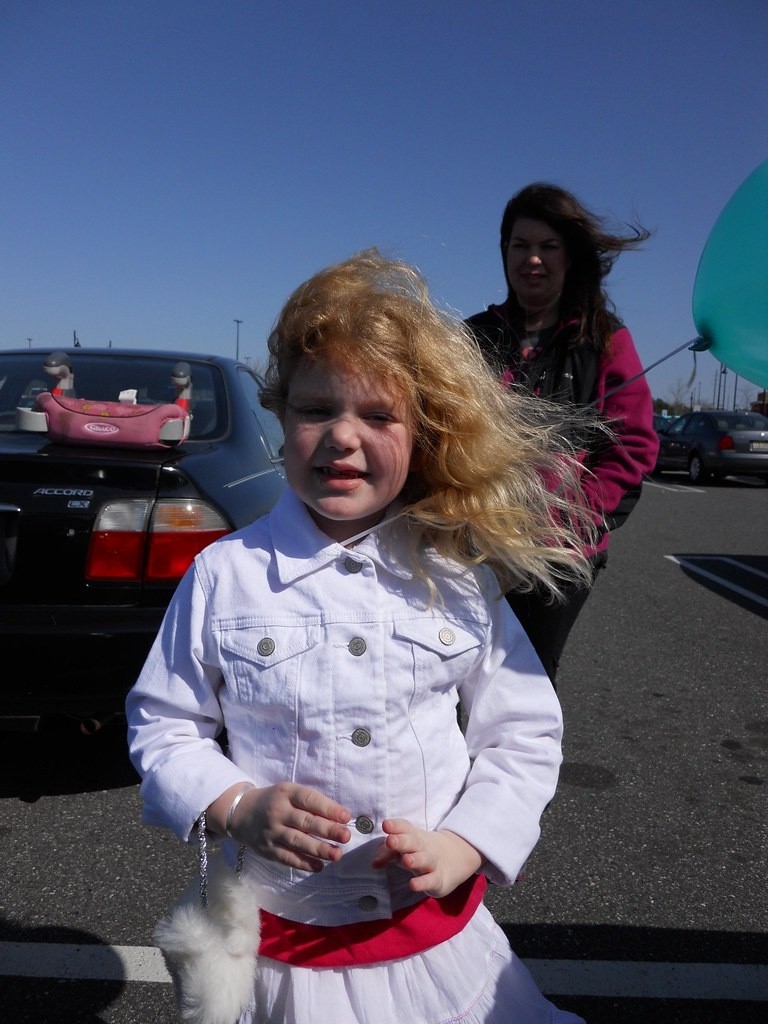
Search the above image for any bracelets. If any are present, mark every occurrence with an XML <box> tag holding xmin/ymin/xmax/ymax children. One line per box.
<box><xmin>197</xmin><ymin>781</ymin><xmax>254</xmax><ymax>913</ymax></box>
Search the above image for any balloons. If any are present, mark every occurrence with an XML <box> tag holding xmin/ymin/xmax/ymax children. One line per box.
<box><xmin>686</xmin><ymin>157</ymin><xmax>768</xmax><ymax>388</ymax></box>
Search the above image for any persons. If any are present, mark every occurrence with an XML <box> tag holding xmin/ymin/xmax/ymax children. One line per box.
<box><xmin>458</xmin><ymin>182</ymin><xmax>660</xmax><ymax>691</ymax></box>
<box><xmin>126</xmin><ymin>252</ymin><xmax>587</xmax><ymax>1024</ymax></box>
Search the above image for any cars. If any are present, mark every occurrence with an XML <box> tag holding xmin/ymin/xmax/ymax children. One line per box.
<box><xmin>653</xmin><ymin>410</ymin><xmax>768</xmax><ymax>485</ymax></box>
<box><xmin>653</xmin><ymin>412</ymin><xmax>672</xmax><ymax>435</ymax></box>
<box><xmin>1</xmin><ymin>347</ymin><xmax>291</xmax><ymax>737</ymax></box>
